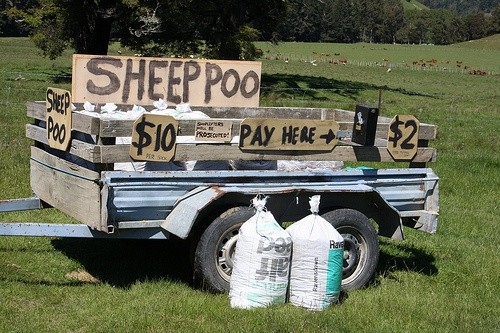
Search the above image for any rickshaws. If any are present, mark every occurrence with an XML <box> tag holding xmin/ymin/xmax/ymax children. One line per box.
<box><xmin>0</xmin><ymin>86</ymin><xmax>442</xmax><ymax>297</ymax></box>
